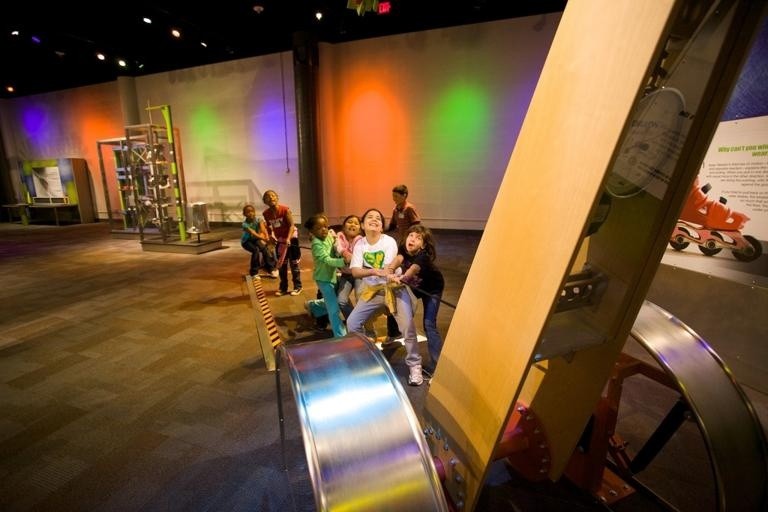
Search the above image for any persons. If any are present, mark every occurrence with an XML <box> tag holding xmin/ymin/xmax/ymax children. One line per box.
<box><xmin>304</xmin><ymin>184</ymin><xmax>445</xmax><ymax>386</ymax></box>
<box><xmin>262</xmin><ymin>189</ymin><xmax>303</xmax><ymax>297</ymax></box>
<box><xmin>239</xmin><ymin>205</ymin><xmax>279</xmax><ymax>280</ymax></box>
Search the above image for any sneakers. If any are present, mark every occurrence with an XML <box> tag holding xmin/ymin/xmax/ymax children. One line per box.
<box><xmin>275</xmin><ymin>288</ymin><xmax>301</xmax><ymax>295</ymax></box>
<box><xmin>254</xmin><ymin>274</ymin><xmax>261</xmax><ymax>279</ymax></box>
<box><xmin>408</xmin><ymin>365</ymin><xmax>424</xmax><ymax>385</ymax></box>
<box><xmin>267</xmin><ymin>271</ymin><xmax>278</xmax><ymax>277</ymax></box>
